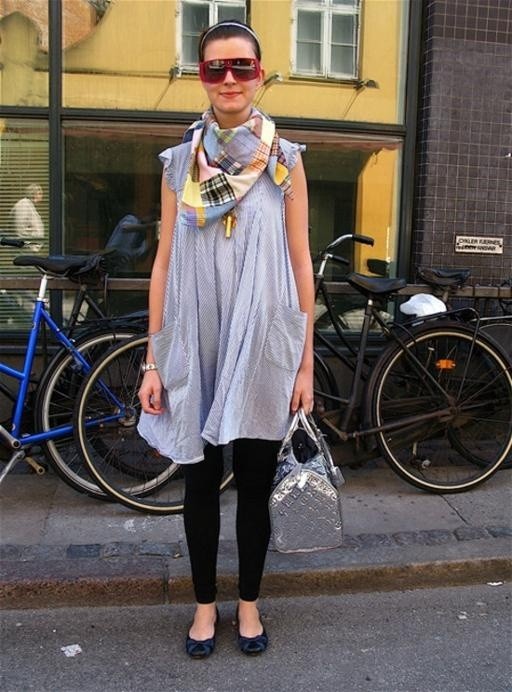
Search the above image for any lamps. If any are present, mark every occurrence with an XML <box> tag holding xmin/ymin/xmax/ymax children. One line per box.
<box><xmin>355</xmin><ymin>76</ymin><xmax>383</xmax><ymax>92</ymax></box>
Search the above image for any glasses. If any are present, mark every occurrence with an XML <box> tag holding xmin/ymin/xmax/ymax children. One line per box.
<box><xmin>199</xmin><ymin>57</ymin><xmax>262</xmax><ymax>85</ymax></box>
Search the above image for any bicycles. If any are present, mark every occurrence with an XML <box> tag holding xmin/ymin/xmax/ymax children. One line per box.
<box><xmin>0</xmin><ymin>256</ymin><xmax>183</xmax><ymax>505</ymax></box>
<box><xmin>0</xmin><ymin>237</ymin><xmax>512</xmax><ymax>482</ymax></box>
<box><xmin>73</xmin><ymin>226</ymin><xmax>512</xmax><ymax>516</ymax></box>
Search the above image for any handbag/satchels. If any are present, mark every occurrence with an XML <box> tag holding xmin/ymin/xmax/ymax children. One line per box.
<box><xmin>267</xmin><ymin>406</ymin><xmax>346</xmax><ymax>555</ymax></box>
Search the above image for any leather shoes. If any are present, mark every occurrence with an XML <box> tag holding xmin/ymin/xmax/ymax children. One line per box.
<box><xmin>187</xmin><ymin>606</ymin><xmax>220</xmax><ymax>658</ymax></box>
<box><xmin>235</xmin><ymin>603</ymin><xmax>268</xmax><ymax>655</ymax></box>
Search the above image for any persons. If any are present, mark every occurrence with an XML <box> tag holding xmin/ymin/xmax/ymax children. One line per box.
<box><xmin>8</xmin><ymin>182</ymin><xmax>45</xmax><ymax>323</ymax></box>
<box><xmin>134</xmin><ymin>17</ymin><xmax>318</xmax><ymax>660</ymax></box>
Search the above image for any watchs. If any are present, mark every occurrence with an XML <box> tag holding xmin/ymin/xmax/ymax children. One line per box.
<box><xmin>139</xmin><ymin>360</ymin><xmax>158</xmax><ymax>374</ymax></box>
